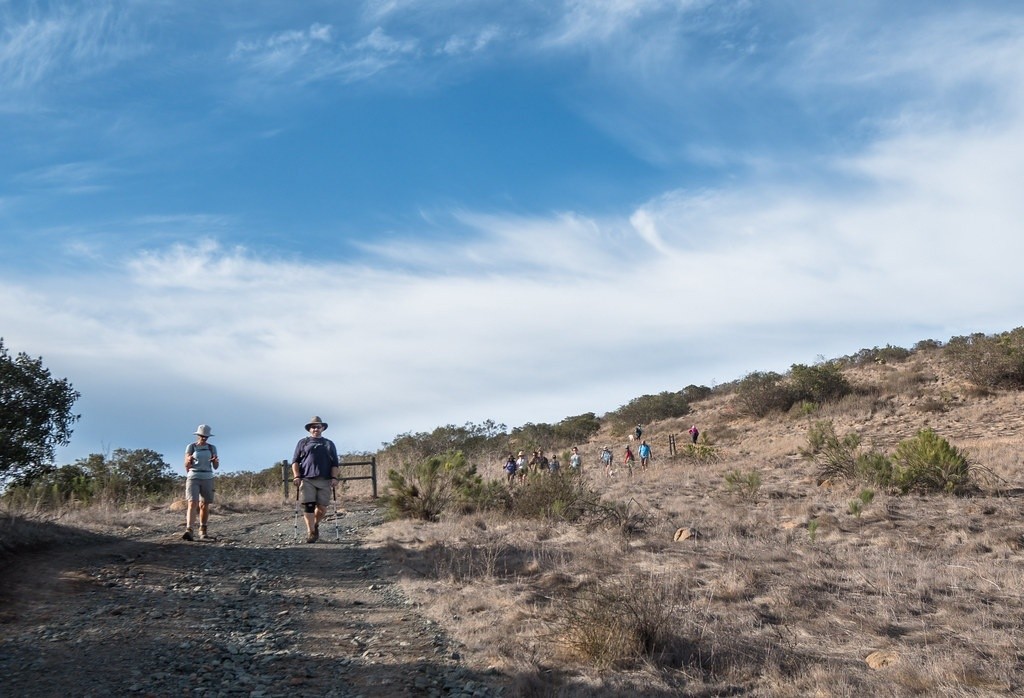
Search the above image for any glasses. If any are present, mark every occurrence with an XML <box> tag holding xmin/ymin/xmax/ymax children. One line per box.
<box><xmin>310</xmin><ymin>426</ymin><xmax>323</xmax><ymax>429</ymax></box>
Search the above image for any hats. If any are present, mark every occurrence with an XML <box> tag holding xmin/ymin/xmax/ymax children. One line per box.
<box><xmin>305</xmin><ymin>416</ymin><xmax>328</xmax><ymax>432</ymax></box>
<box><xmin>193</xmin><ymin>424</ymin><xmax>215</xmax><ymax>437</ymax></box>
<box><xmin>519</xmin><ymin>451</ymin><xmax>525</xmax><ymax>457</ymax></box>
<box><xmin>508</xmin><ymin>455</ymin><xmax>515</xmax><ymax>459</ymax></box>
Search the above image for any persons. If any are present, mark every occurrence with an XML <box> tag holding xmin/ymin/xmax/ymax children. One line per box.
<box><xmin>292</xmin><ymin>415</ymin><xmax>338</xmax><ymax>543</ymax></box>
<box><xmin>599</xmin><ymin>446</ymin><xmax>613</xmax><ymax>467</ymax></box>
<box><xmin>549</xmin><ymin>455</ymin><xmax>560</xmax><ymax>472</ymax></box>
<box><xmin>635</xmin><ymin>424</ymin><xmax>642</xmax><ymax>440</ymax></box>
<box><xmin>623</xmin><ymin>445</ymin><xmax>634</xmax><ymax>478</ymax></box>
<box><xmin>570</xmin><ymin>447</ymin><xmax>582</xmax><ymax>467</ymax></box>
<box><xmin>503</xmin><ymin>450</ymin><xmax>549</xmax><ymax>484</ymax></box>
<box><xmin>638</xmin><ymin>440</ymin><xmax>653</xmax><ymax>473</ymax></box>
<box><xmin>688</xmin><ymin>425</ymin><xmax>699</xmax><ymax>444</ymax></box>
<box><xmin>182</xmin><ymin>425</ymin><xmax>220</xmax><ymax>541</ymax></box>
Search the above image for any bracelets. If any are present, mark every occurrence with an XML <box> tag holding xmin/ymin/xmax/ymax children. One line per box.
<box><xmin>294</xmin><ymin>476</ymin><xmax>301</xmax><ymax>479</ymax></box>
<box><xmin>332</xmin><ymin>477</ymin><xmax>337</xmax><ymax>479</ymax></box>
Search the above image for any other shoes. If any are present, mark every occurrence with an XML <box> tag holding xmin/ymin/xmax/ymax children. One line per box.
<box><xmin>315</xmin><ymin>525</ymin><xmax>319</xmax><ymax>539</ymax></box>
<box><xmin>198</xmin><ymin>525</ymin><xmax>208</xmax><ymax>539</ymax></box>
<box><xmin>307</xmin><ymin>531</ymin><xmax>315</xmax><ymax>543</ymax></box>
<box><xmin>183</xmin><ymin>528</ymin><xmax>193</xmax><ymax>541</ymax></box>
